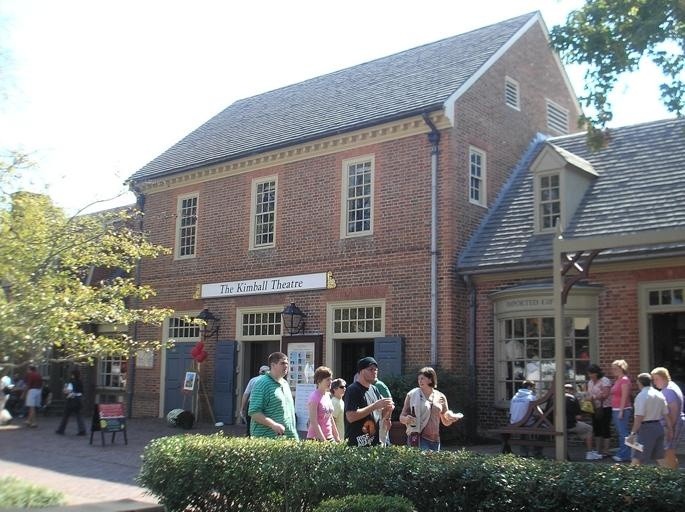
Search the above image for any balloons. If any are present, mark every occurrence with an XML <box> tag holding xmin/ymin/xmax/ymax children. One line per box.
<box><xmin>191</xmin><ymin>342</ymin><xmax>208</xmax><ymax>363</ymax></box>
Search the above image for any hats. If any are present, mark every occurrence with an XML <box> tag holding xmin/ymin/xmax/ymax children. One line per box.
<box><xmin>358</xmin><ymin>357</ymin><xmax>377</xmax><ymax>369</ymax></box>
<box><xmin>259</xmin><ymin>366</ymin><xmax>269</xmax><ymax>374</ymax></box>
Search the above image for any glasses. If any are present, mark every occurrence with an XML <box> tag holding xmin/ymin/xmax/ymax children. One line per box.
<box><xmin>340</xmin><ymin>385</ymin><xmax>347</xmax><ymax>389</ymax></box>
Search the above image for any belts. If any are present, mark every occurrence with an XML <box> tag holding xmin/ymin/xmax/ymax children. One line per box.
<box><xmin>643</xmin><ymin>420</ymin><xmax>659</xmax><ymax>424</ymax></box>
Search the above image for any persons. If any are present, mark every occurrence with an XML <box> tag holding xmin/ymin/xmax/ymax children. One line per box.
<box><xmin>239</xmin><ymin>350</ymin><xmax>467</xmax><ymax>452</ymax></box>
<box><xmin>0</xmin><ymin>349</ymin><xmax>91</xmax><ymax>436</ymax></box>
<box><xmin>506</xmin><ymin>351</ymin><xmax>685</xmax><ymax>471</ymax></box>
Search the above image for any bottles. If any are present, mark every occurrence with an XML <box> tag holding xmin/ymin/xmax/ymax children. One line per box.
<box><xmin>410</xmin><ymin>406</ymin><xmax>416</xmax><ymax>427</ymax></box>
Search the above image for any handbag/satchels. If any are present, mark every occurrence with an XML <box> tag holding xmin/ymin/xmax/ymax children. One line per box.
<box><xmin>63</xmin><ymin>382</ymin><xmax>73</xmax><ymax>394</ymax></box>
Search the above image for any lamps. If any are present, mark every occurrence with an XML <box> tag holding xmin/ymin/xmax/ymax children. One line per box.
<box><xmin>281</xmin><ymin>303</ymin><xmax>306</xmax><ymax>336</ymax></box>
<box><xmin>196</xmin><ymin>309</ymin><xmax>220</xmax><ymax>342</ymax></box>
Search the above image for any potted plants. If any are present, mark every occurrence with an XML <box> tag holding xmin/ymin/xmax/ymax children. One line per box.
<box><xmin>384</xmin><ymin>378</ymin><xmax>416</xmax><ymax>445</ymax></box>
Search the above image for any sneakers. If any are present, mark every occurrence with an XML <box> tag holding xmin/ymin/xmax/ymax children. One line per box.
<box><xmin>584</xmin><ymin>450</ymin><xmax>623</xmax><ymax>461</ymax></box>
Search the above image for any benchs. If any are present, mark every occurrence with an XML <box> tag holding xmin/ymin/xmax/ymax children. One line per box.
<box><xmin>486</xmin><ymin>375</ymin><xmax>575</xmax><ymax>460</ymax></box>
